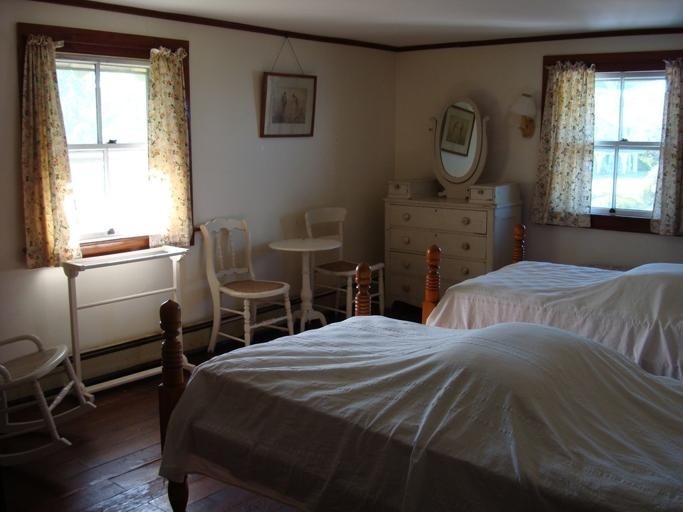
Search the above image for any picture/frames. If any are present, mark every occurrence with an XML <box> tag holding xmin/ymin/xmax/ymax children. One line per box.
<box><xmin>258</xmin><ymin>69</ymin><xmax>317</xmax><ymax>137</ymax></box>
<box><xmin>439</xmin><ymin>104</ymin><xmax>475</xmax><ymax>156</ymax></box>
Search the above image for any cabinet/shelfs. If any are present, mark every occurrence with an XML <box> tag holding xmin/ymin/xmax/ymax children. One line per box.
<box><xmin>383</xmin><ymin>179</ymin><xmax>523</xmax><ymax>309</ymax></box>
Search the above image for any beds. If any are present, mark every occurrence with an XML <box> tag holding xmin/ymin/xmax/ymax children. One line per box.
<box><xmin>155</xmin><ymin>260</ymin><xmax>683</xmax><ymax>512</ymax></box>
<box><xmin>422</xmin><ymin>222</ymin><xmax>683</xmax><ymax>380</ymax></box>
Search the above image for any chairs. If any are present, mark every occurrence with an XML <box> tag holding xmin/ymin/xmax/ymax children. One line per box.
<box><xmin>0</xmin><ymin>334</ymin><xmax>99</xmax><ymax>464</ymax></box>
<box><xmin>199</xmin><ymin>216</ymin><xmax>295</xmax><ymax>353</ymax></box>
<box><xmin>304</xmin><ymin>205</ymin><xmax>385</xmax><ymax>317</ymax></box>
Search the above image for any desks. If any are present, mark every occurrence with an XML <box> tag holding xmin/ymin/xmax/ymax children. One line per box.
<box><xmin>267</xmin><ymin>236</ymin><xmax>342</xmax><ymax>331</ymax></box>
<box><xmin>61</xmin><ymin>243</ymin><xmax>198</xmax><ymax>404</ymax></box>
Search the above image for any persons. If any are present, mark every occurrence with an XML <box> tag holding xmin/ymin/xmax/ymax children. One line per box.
<box><xmin>281</xmin><ymin>91</ymin><xmax>287</xmax><ymax>114</ymax></box>
<box><xmin>448</xmin><ymin>117</ymin><xmax>466</xmax><ymax>141</ymax></box>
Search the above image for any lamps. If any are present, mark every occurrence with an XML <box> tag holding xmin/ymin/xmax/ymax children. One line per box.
<box><xmin>508</xmin><ymin>92</ymin><xmax>538</xmax><ymax>138</ymax></box>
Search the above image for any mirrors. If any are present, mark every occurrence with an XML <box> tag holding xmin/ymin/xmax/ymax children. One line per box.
<box><xmin>427</xmin><ymin>96</ymin><xmax>491</xmax><ymax>183</ymax></box>
